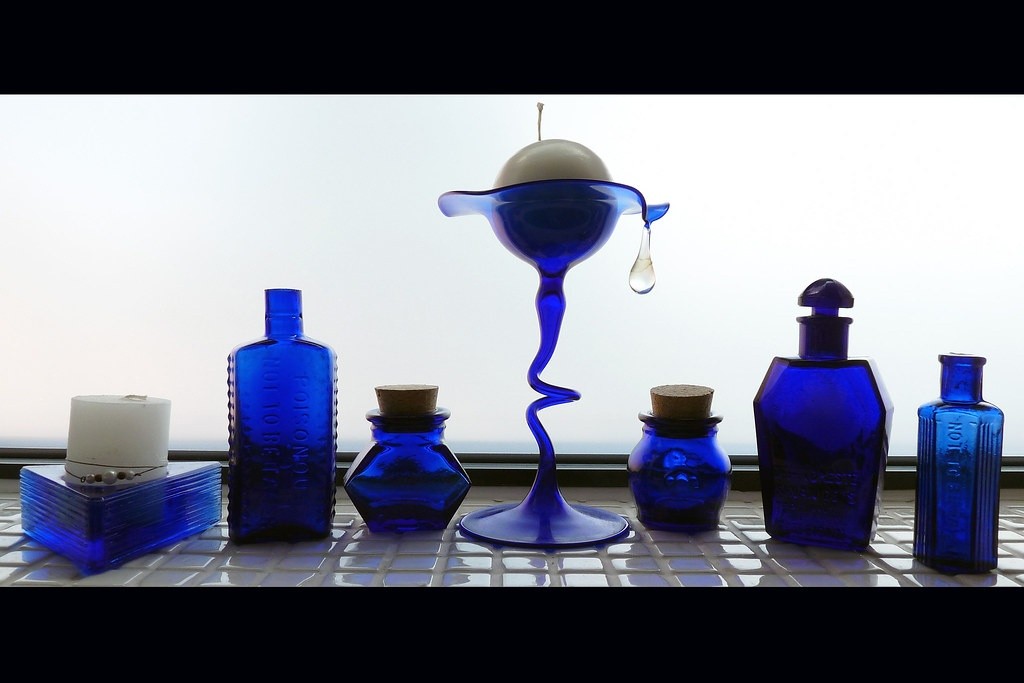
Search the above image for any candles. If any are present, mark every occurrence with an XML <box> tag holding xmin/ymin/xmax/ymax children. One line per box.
<box><xmin>64</xmin><ymin>395</ymin><xmax>171</xmax><ymax>488</ymax></box>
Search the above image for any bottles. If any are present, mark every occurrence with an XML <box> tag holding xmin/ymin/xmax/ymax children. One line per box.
<box><xmin>343</xmin><ymin>385</ymin><xmax>472</xmax><ymax>535</ymax></box>
<box><xmin>227</xmin><ymin>288</ymin><xmax>339</xmax><ymax>546</ymax></box>
<box><xmin>914</xmin><ymin>354</ymin><xmax>1004</xmax><ymax>575</ymax></box>
<box><xmin>628</xmin><ymin>385</ymin><xmax>731</xmax><ymax>532</ymax></box>
<box><xmin>753</xmin><ymin>279</ymin><xmax>894</xmax><ymax>553</ymax></box>
